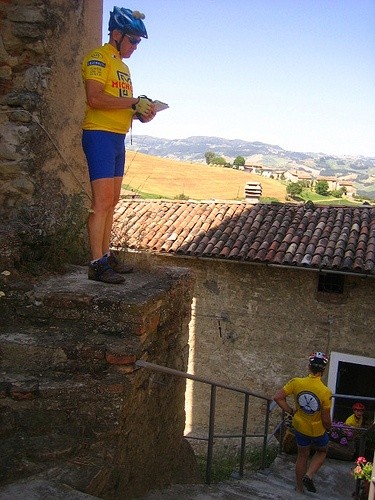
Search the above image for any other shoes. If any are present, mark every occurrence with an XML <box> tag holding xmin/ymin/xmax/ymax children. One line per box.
<box><xmin>302</xmin><ymin>476</ymin><xmax>317</xmax><ymax>493</ymax></box>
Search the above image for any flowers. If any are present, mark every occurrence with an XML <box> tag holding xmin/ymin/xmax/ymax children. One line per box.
<box><xmin>331</xmin><ymin>419</ymin><xmax>352</xmax><ymax>446</ymax></box>
<box><xmin>349</xmin><ymin>456</ymin><xmax>372</xmax><ymax>482</ymax></box>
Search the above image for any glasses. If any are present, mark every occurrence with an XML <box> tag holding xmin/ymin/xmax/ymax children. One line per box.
<box><xmin>120</xmin><ymin>32</ymin><xmax>142</xmax><ymax>45</ymax></box>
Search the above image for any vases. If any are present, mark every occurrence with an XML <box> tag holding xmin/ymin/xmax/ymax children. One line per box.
<box><xmin>355</xmin><ymin>479</ymin><xmax>368</xmax><ymax>499</ymax></box>
<box><xmin>326</xmin><ymin>439</ymin><xmax>355</xmax><ymax>461</ymax></box>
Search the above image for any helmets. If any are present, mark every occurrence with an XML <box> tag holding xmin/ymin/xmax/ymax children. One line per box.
<box><xmin>108</xmin><ymin>5</ymin><xmax>148</xmax><ymax>39</ymax></box>
<box><xmin>310</xmin><ymin>351</ymin><xmax>328</xmax><ymax>367</ymax></box>
<box><xmin>352</xmin><ymin>403</ymin><xmax>365</xmax><ymax>410</ymax></box>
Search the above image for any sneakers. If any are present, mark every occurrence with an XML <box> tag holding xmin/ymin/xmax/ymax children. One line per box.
<box><xmin>87</xmin><ymin>260</ymin><xmax>125</xmax><ymax>283</ymax></box>
<box><xmin>106</xmin><ymin>256</ymin><xmax>134</xmax><ymax>274</ymax></box>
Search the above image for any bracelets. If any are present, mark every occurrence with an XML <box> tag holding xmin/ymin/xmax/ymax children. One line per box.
<box><xmin>290</xmin><ymin>408</ymin><xmax>293</xmax><ymax>415</ymax></box>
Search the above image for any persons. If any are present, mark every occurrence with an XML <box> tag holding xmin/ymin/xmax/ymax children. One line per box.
<box><xmin>82</xmin><ymin>6</ymin><xmax>156</xmax><ymax>284</ymax></box>
<box><xmin>343</xmin><ymin>402</ymin><xmax>365</xmax><ymax>428</ymax></box>
<box><xmin>274</xmin><ymin>351</ymin><xmax>332</xmax><ymax>493</ymax></box>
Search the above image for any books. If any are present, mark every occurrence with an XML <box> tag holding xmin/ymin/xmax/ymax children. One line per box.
<box><xmin>154</xmin><ymin>100</ymin><xmax>169</xmax><ymax>112</ymax></box>
